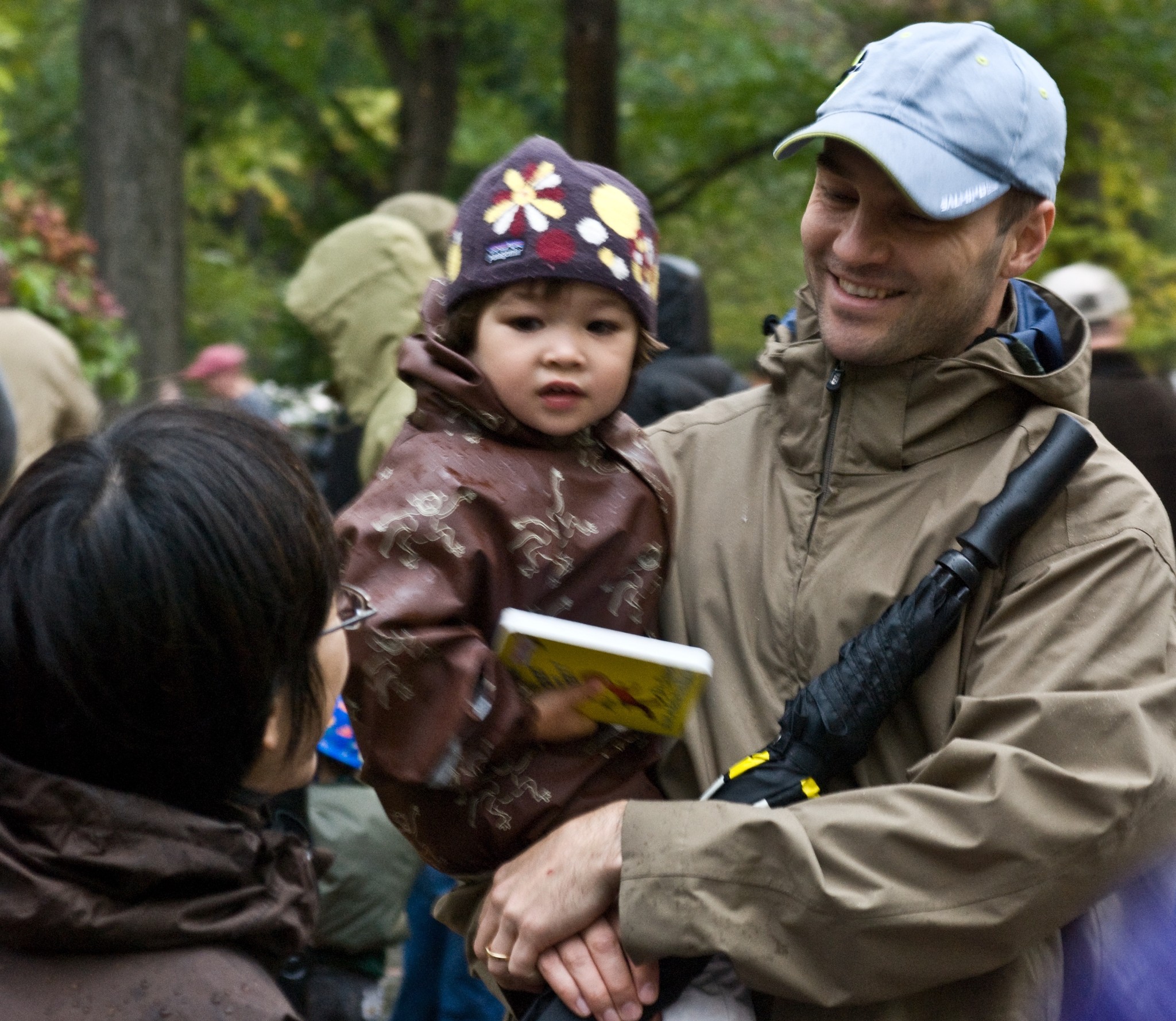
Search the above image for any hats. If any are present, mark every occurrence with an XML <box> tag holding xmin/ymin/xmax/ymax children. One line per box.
<box><xmin>186</xmin><ymin>344</ymin><xmax>244</xmax><ymax>379</ymax></box>
<box><xmin>774</xmin><ymin>20</ymin><xmax>1067</xmax><ymax>222</ymax></box>
<box><xmin>447</xmin><ymin>134</ymin><xmax>658</xmax><ymax>330</ymax></box>
<box><xmin>1039</xmin><ymin>260</ymin><xmax>1131</xmax><ymax>322</ymax></box>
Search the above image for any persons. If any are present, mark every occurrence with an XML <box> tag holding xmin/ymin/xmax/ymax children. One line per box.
<box><xmin>334</xmin><ymin>136</ymin><xmax>711</xmax><ymax>1021</ymax></box>
<box><xmin>0</xmin><ymin>19</ymin><xmax>1176</xmax><ymax>1021</ymax></box>
<box><xmin>470</xmin><ymin>20</ymin><xmax>1176</xmax><ymax>1021</ymax></box>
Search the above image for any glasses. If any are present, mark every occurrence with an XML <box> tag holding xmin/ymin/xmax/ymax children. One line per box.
<box><xmin>313</xmin><ymin>581</ymin><xmax>376</xmax><ymax>637</ymax></box>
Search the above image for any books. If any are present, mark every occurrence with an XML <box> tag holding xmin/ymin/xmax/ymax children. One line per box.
<box><xmin>491</xmin><ymin>599</ymin><xmax>713</xmax><ymax>737</ymax></box>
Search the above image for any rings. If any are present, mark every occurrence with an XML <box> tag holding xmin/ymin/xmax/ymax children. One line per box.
<box><xmin>485</xmin><ymin>948</ymin><xmax>509</xmax><ymax>962</ymax></box>
